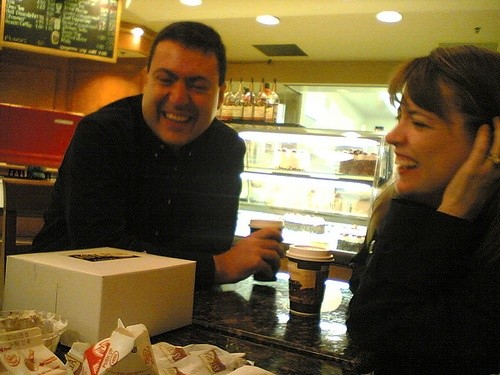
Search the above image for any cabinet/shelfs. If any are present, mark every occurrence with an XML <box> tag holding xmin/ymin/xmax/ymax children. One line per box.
<box><xmin>222</xmin><ymin>122</ymin><xmax>398</xmax><ymax>283</ymax></box>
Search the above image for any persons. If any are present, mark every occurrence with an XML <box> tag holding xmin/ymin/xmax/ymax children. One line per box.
<box><xmin>31</xmin><ymin>21</ymin><xmax>286</xmax><ymax>288</ymax></box>
<box><xmin>347</xmin><ymin>44</ymin><xmax>500</xmax><ymax>375</ymax></box>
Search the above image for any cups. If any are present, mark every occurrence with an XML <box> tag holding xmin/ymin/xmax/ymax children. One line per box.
<box><xmin>285</xmin><ymin>243</ymin><xmax>335</xmax><ymax>315</ymax></box>
<box><xmin>248</xmin><ymin>219</ymin><xmax>285</xmax><ymax>282</ymax></box>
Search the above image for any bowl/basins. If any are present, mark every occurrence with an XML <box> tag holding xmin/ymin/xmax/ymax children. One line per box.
<box><xmin>0</xmin><ymin>310</ymin><xmax>68</xmax><ymax>353</ymax></box>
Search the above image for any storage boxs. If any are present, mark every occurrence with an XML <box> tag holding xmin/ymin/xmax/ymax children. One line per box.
<box><xmin>1</xmin><ymin>246</ymin><xmax>196</xmax><ymax>348</ymax></box>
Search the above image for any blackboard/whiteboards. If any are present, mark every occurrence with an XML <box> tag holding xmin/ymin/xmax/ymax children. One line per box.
<box><xmin>0</xmin><ymin>0</ymin><xmax>122</xmax><ymax>63</ymax></box>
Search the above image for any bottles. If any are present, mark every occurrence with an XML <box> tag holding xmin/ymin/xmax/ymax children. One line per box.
<box><xmin>216</xmin><ymin>77</ymin><xmax>280</xmax><ymax>123</ymax></box>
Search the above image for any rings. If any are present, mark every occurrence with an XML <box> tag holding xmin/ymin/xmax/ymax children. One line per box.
<box><xmin>488</xmin><ymin>156</ymin><xmax>500</xmax><ymax>163</ymax></box>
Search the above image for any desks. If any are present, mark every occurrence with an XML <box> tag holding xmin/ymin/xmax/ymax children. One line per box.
<box><xmin>50</xmin><ymin>271</ymin><xmax>376</xmax><ymax>375</ymax></box>
